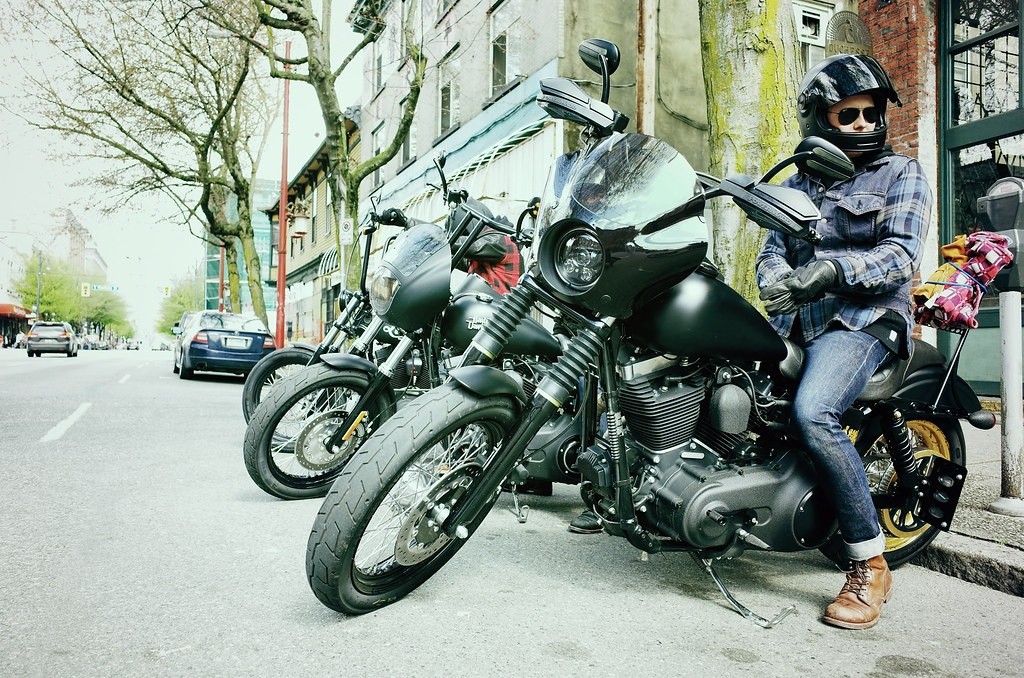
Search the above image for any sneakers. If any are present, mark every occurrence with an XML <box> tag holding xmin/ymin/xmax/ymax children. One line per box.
<box><xmin>821</xmin><ymin>552</ymin><xmax>892</xmax><ymax>629</ymax></box>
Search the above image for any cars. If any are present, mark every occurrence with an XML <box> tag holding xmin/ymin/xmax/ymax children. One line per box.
<box><xmin>151</xmin><ymin>343</ymin><xmax>165</xmax><ymax>351</ymax></box>
<box><xmin>27</xmin><ymin>322</ymin><xmax>78</xmax><ymax>358</ymax></box>
<box><xmin>75</xmin><ymin>335</ymin><xmax>107</xmax><ymax>350</ymax></box>
<box><xmin>127</xmin><ymin>341</ymin><xmax>139</xmax><ymax>350</ymax></box>
<box><xmin>170</xmin><ymin>310</ymin><xmax>276</xmax><ymax>383</ymax></box>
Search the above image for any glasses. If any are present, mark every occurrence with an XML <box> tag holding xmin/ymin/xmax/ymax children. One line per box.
<box><xmin>827</xmin><ymin>106</ymin><xmax>882</xmax><ymax>126</ymax></box>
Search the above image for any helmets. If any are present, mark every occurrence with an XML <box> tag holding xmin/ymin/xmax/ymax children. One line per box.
<box><xmin>445</xmin><ymin>198</ymin><xmax>495</xmax><ymax>236</ymax></box>
<box><xmin>797</xmin><ymin>53</ymin><xmax>895</xmax><ymax>152</ymax></box>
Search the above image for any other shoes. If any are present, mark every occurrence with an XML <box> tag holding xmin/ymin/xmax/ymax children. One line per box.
<box><xmin>568</xmin><ymin>509</ymin><xmax>604</xmax><ymax>533</ymax></box>
<box><xmin>502</xmin><ymin>476</ymin><xmax>552</xmax><ymax>496</ymax></box>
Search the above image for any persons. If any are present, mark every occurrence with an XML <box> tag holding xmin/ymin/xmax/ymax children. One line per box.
<box><xmin>379</xmin><ymin>195</ymin><xmax>529</xmax><ymax>337</ymax></box>
<box><xmin>493</xmin><ymin>162</ymin><xmax>615</xmax><ymax>534</ymax></box>
<box><xmin>757</xmin><ymin>55</ymin><xmax>933</xmax><ymax>630</ymax></box>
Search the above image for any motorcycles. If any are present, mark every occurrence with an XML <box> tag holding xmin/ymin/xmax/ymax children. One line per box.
<box><xmin>305</xmin><ymin>38</ymin><xmax>997</xmax><ymax>629</ymax></box>
<box><xmin>242</xmin><ymin>149</ymin><xmax>600</xmax><ymax>523</ymax></box>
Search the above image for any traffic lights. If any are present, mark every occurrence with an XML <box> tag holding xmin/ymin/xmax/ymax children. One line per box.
<box><xmin>165</xmin><ymin>287</ymin><xmax>168</xmax><ymax>296</ymax></box>
<box><xmin>84</xmin><ymin>285</ymin><xmax>88</xmax><ymax>295</ymax></box>
<box><xmin>112</xmin><ymin>287</ymin><xmax>119</xmax><ymax>291</ymax></box>
<box><xmin>92</xmin><ymin>286</ymin><xmax>98</xmax><ymax>290</ymax></box>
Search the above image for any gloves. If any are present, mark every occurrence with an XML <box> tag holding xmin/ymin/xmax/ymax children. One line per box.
<box><xmin>759</xmin><ymin>259</ymin><xmax>836</xmax><ymax>318</ymax></box>
<box><xmin>379</xmin><ymin>207</ymin><xmax>407</xmax><ymax>229</ymax></box>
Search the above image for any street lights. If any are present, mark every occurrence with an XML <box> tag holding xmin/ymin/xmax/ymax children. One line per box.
<box><xmin>37</xmin><ymin>250</ymin><xmax>51</xmax><ymax>315</ymax></box>
<box><xmin>207</xmin><ymin>30</ymin><xmax>291</xmax><ymax>350</ymax></box>
